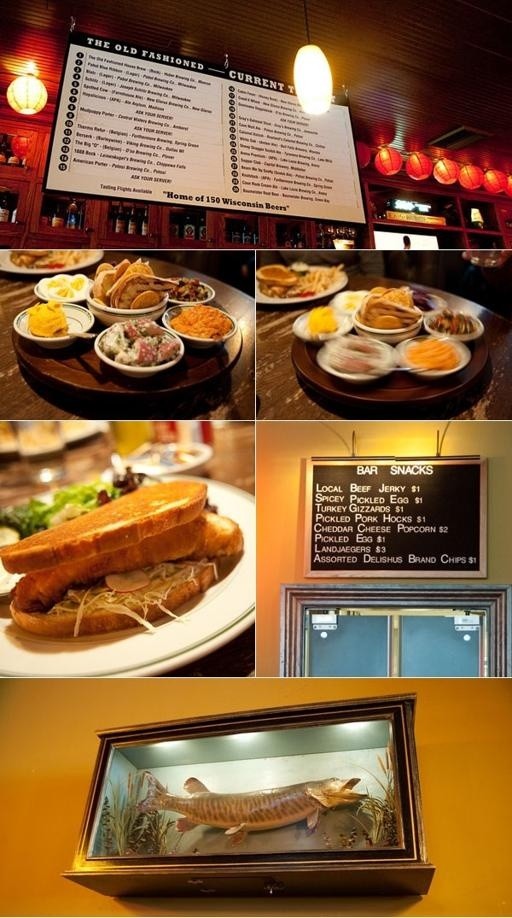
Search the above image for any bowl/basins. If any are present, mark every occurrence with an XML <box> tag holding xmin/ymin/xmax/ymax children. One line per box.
<box><xmin>12</xmin><ymin>277</ymin><xmax>238</xmax><ymax>379</ymax></box>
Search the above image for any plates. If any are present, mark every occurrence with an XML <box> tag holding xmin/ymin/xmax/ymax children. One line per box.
<box><xmin>256</xmin><ymin>263</ymin><xmax>486</xmax><ymax>385</ymax></box>
<box><xmin>0</xmin><ymin>421</ymin><xmax>255</xmax><ymax>677</ymax></box>
<box><xmin>0</xmin><ymin>249</ymin><xmax>104</xmax><ymax>274</ymax></box>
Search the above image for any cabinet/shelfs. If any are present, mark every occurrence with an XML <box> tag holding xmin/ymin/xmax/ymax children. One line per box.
<box><xmin>365</xmin><ymin>182</ymin><xmax>512</xmax><ymax>248</ymax></box>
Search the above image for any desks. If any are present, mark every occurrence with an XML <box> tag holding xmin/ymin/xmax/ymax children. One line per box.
<box><xmin>0</xmin><ymin>251</ymin><xmax>255</xmax><ymax>420</ymax></box>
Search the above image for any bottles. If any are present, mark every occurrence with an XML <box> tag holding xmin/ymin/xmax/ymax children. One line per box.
<box><xmin>0</xmin><ymin>192</ymin><xmax>359</xmax><ymax>249</ymax></box>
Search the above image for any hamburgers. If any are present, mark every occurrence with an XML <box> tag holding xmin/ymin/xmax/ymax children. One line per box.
<box><xmin>0</xmin><ymin>479</ymin><xmax>244</xmax><ymax>638</ymax></box>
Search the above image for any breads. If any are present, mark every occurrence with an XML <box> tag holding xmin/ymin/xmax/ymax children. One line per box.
<box><xmin>257</xmin><ymin>264</ymin><xmax>296</xmax><ymax>286</ymax></box>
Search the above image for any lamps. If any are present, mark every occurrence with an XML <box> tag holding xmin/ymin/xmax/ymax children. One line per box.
<box><xmin>375</xmin><ymin>144</ymin><xmax>512</xmax><ymax>197</ymax></box>
<box><xmin>292</xmin><ymin>1</ymin><xmax>333</xmax><ymax>115</ymax></box>
<box><xmin>6</xmin><ymin>71</ymin><xmax>48</xmax><ymax>115</ymax></box>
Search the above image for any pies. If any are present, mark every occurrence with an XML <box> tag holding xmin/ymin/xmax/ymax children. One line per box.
<box><xmin>92</xmin><ymin>260</ymin><xmax>179</xmax><ymax>309</ymax></box>
<box><xmin>355</xmin><ymin>286</ymin><xmax>421</xmax><ymax>329</ymax></box>
